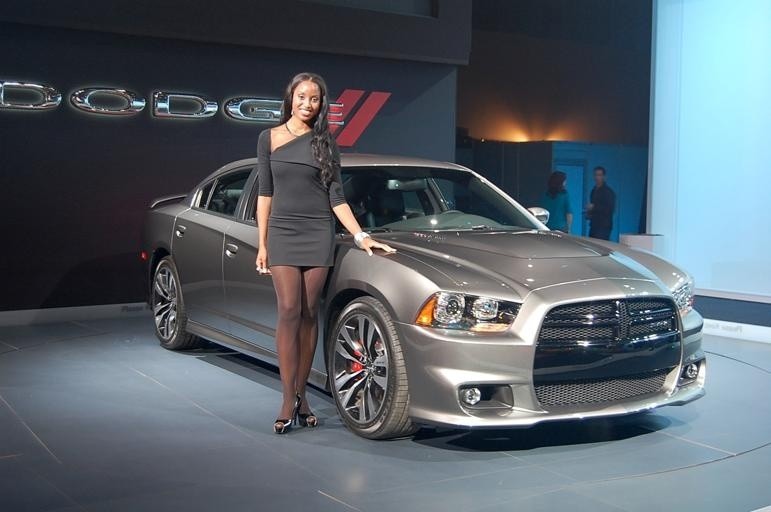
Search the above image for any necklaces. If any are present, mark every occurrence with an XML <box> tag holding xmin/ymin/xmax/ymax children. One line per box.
<box><xmin>285</xmin><ymin>121</ymin><xmax>306</xmax><ymax>140</ymax></box>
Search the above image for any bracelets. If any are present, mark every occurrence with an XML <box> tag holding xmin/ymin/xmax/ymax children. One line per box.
<box><xmin>352</xmin><ymin>231</ymin><xmax>373</xmax><ymax>244</ymax></box>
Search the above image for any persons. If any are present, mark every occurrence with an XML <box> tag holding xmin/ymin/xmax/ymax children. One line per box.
<box><xmin>256</xmin><ymin>69</ymin><xmax>396</xmax><ymax>433</ymax></box>
<box><xmin>583</xmin><ymin>165</ymin><xmax>617</xmax><ymax>241</ymax></box>
<box><xmin>540</xmin><ymin>168</ymin><xmax>573</xmax><ymax>239</ymax></box>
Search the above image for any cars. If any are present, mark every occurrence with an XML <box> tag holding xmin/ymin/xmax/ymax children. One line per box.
<box><xmin>138</xmin><ymin>149</ymin><xmax>710</xmax><ymax>442</ymax></box>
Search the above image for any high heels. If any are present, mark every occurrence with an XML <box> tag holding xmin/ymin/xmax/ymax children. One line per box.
<box><xmin>273</xmin><ymin>394</ymin><xmax>317</xmax><ymax>435</ymax></box>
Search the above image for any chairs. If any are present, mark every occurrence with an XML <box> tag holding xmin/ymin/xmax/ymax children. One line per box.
<box><xmin>364</xmin><ymin>174</ymin><xmax>408</xmax><ymax>225</ymax></box>
